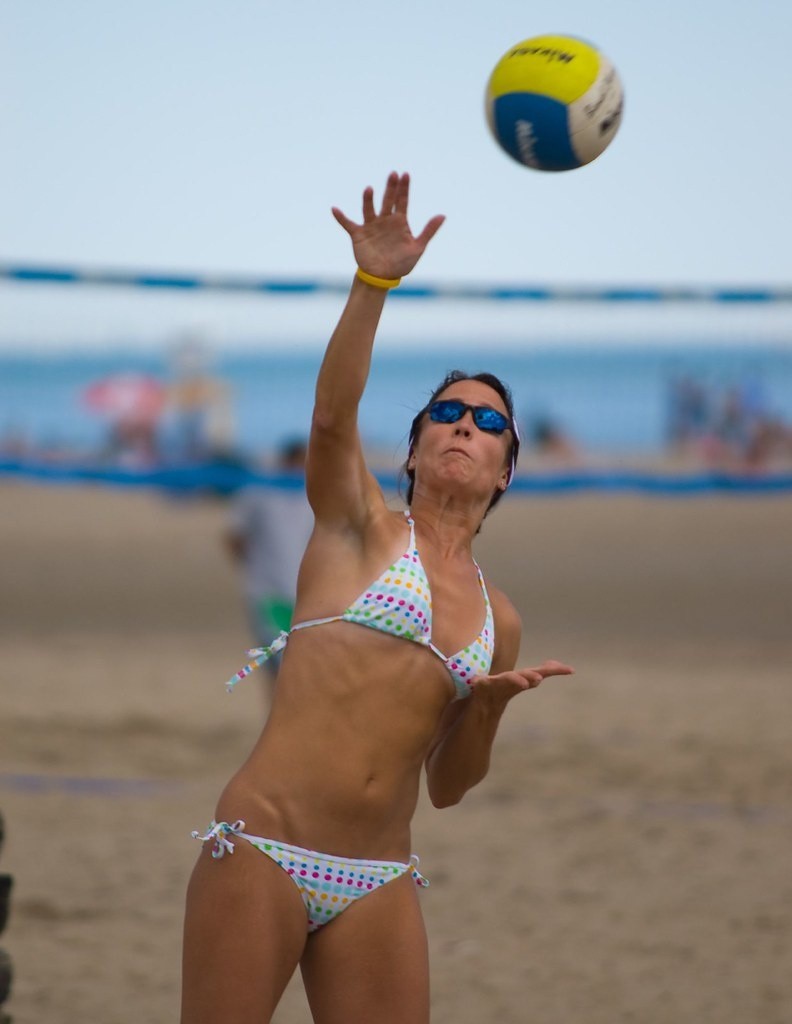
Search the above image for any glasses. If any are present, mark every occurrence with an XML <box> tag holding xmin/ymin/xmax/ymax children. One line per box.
<box><xmin>427</xmin><ymin>400</ymin><xmax>511</xmax><ymax>435</ymax></box>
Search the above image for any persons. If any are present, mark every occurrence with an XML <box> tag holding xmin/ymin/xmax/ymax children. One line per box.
<box><xmin>181</xmin><ymin>173</ymin><xmax>577</xmax><ymax>1023</ymax></box>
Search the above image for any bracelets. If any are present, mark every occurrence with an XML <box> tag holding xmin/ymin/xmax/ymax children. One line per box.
<box><xmin>354</xmin><ymin>264</ymin><xmax>403</xmax><ymax>292</ymax></box>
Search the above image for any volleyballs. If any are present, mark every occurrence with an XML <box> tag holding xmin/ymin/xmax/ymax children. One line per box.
<box><xmin>480</xmin><ymin>30</ymin><xmax>627</xmax><ymax>173</ymax></box>
